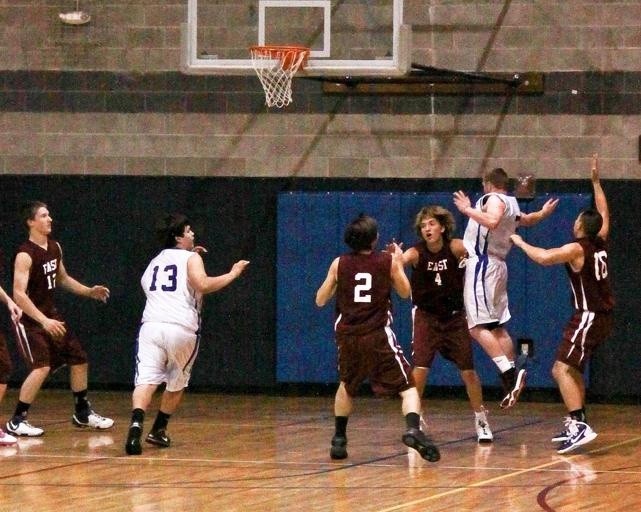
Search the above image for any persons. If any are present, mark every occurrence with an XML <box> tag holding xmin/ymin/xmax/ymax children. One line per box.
<box><xmin>0</xmin><ymin>284</ymin><xmax>22</xmax><ymax>445</ymax></box>
<box><xmin>453</xmin><ymin>168</ymin><xmax>557</xmax><ymax>409</ymax></box>
<box><xmin>508</xmin><ymin>152</ymin><xmax>612</xmax><ymax>455</ymax></box>
<box><xmin>4</xmin><ymin>200</ymin><xmax>115</xmax><ymax>438</ymax></box>
<box><xmin>382</xmin><ymin>204</ymin><xmax>495</xmax><ymax>446</ymax></box>
<box><xmin>315</xmin><ymin>212</ymin><xmax>440</xmax><ymax>462</ymax></box>
<box><xmin>125</xmin><ymin>216</ymin><xmax>251</xmax><ymax>455</ymax></box>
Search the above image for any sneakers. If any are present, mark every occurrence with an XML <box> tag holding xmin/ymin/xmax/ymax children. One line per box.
<box><xmin>1</xmin><ymin>428</ymin><xmax>18</xmax><ymax>445</ymax></box>
<box><xmin>5</xmin><ymin>411</ymin><xmax>45</xmax><ymax>437</ymax></box>
<box><xmin>125</xmin><ymin>419</ymin><xmax>144</xmax><ymax>455</ymax></box>
<box><xmin>402</xmin><ymin>427</ymin><xmax>441</xmax><ymax>463</ymax></box>
<box><xmin>550</xmin><ymin>426</ymin><xmax>571</xmax><ymax>443</ymax></box>
<box><xmin>474</xmin><ymin>416</ymin><xmax>493</xmax><ymax>443</ymax></box>
<box><xmin>146</xmin><ymin>428</ymin><xmax>171</xmax><ymax>447</ymax></box>
<box><xmin>555</xmin><ymin>417</ymin><xmax>597</xmax><ymax>454</ymax></box>
<box><xmin>506</xmin><ymin>367</ymin><xmax>527</xmax><ymax>407</ymax></box>
<box><xmin>330</xmin><ymin>437</ymin><xmax>348</xmax><ymax>459</ymax></box>
<box><xmin>499</xmin><ymin>393</ymin><xmax>514</xmax><ymax>411</ymax></box>
<box><xmin>72</xmin><ymin>407</ymin><xmax>115</xmax><ymax>430</ymax></box>
